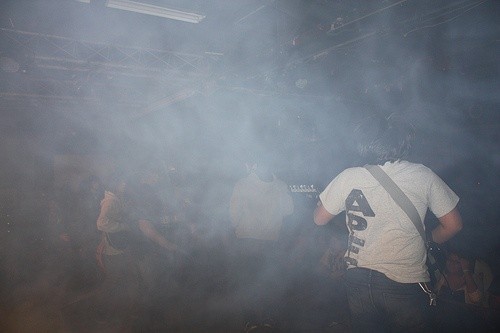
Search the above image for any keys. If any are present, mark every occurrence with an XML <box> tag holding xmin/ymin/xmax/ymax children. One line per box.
<box><xmin>427</xmin><ymin>289</ymin><xmax>440</xmax><ymax>309</ymax></box>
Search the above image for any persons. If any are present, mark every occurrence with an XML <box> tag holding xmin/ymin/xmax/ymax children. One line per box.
<box><xmin>6</xmin><ymin>122</ymin><xmax>348</xmax><ymax>314</ymax></box>
<box><xmin>314</xmin><ymin>113</ymin><xmax>463</xmax><ymax>332</ymax></box>
<box><xmin>424</xmin><ymin>222</ymin><xmax>500</xmax><ymax>333</ymax></box>
